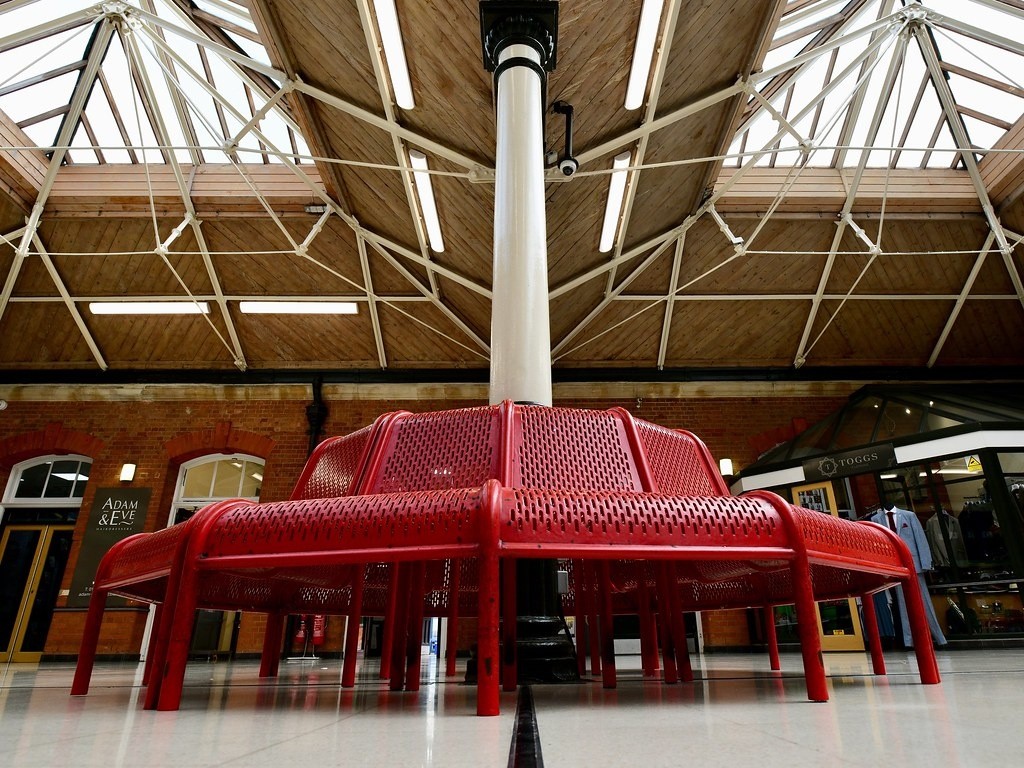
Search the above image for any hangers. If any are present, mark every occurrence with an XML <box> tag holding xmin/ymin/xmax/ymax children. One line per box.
<box><xmin>864</xmin><ymin>503</ymin><xmax>894</xmax><ymax>511</ymax></box>
<box><xmin>935</xmin><ymin>505</ymin><xmax>950</xmax><ymax>516</ymax></box>
<box><xmin>963</xmin><ymin>496</ymin><xmax>986</xmax><ymax>507</ymax></box>
<box><xmin>1011</xmin><ymin>480</ymin><xmax>1024</xmax><ymax>490</ymax></box>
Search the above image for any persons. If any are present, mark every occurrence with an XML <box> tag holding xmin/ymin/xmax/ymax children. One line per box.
<box><xmin>871</xmin><ymin>505</ymin><xmax>948</xmax><ymax>648</ymax></box>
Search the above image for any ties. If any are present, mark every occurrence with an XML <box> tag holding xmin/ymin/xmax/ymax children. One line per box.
<box><xmin>887</xmin><ymin>512</ymin><xmax>897</xmax><ymax>534</ymax></box>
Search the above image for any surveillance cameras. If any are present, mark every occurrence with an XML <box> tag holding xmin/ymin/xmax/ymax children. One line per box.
<box><xmin>560</xmin><ymin>160</ymin><xmax>577</xmax><ymax>176</ymax></box>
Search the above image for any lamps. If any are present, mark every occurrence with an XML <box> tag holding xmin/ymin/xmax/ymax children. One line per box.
<box><xmin>373</xmin><ymin>0</ymin><xmax>416</xmax><ymax>110</ymax></box>
<box><xmin>409</xmin><ymin>148</ymin><xmax>445</xmax><ymax>253</ymax></box>
<box><xmin>119</xmin><ymin>463</ymin><xmax>137</xmax><ymax>482</ymax></box>
<box><xmin>240</xmin><ymin>302</ymin><xmax>360</xmax><ymax>315</ymax></box>
<box><xmin>623</xmin><ymin>0</ymin><xmax>665</xmax><ymax>110</ymax></box>
<box><xmin>89</xmin><ymin>302</ymin><xmax>211</xmax><ymax>314</ymax></box>
<box><xmin>599</xmin><ymin>150</ymin><xmax>632</xmax><ymax>253</ymax></box>
